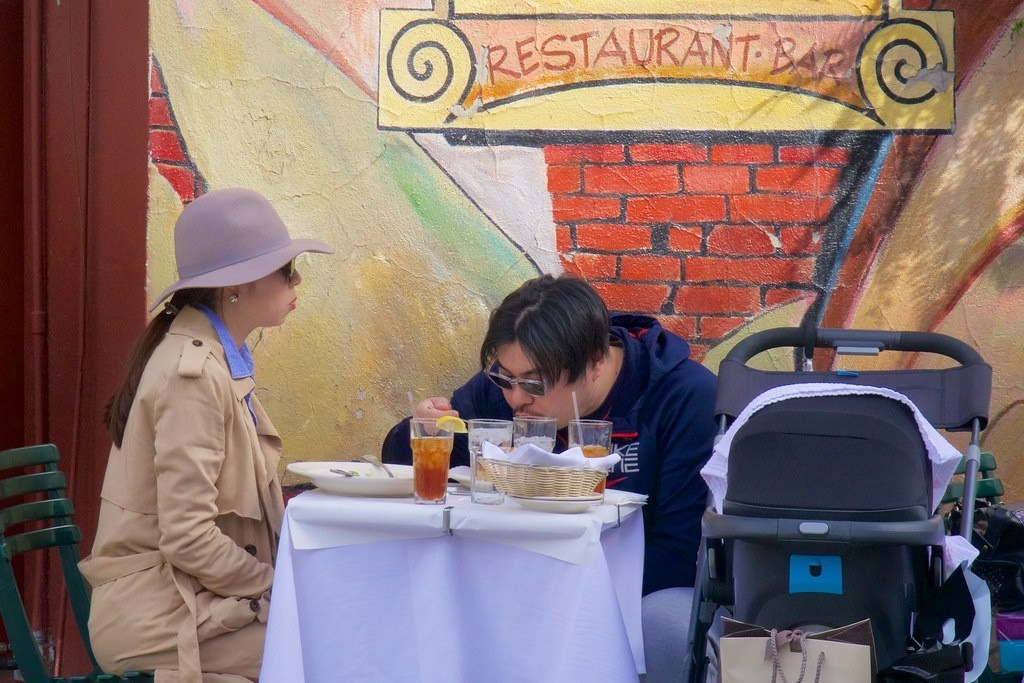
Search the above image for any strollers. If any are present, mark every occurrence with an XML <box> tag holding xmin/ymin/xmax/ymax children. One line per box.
<box><xmin>682</xmin><ymin>326</ymin><xmax>994</xmax><ymax>683</ymax></box>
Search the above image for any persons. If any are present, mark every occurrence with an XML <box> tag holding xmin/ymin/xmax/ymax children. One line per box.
<box><xmin>381</xmin><ymin>274</ymin><xmax>732</xmax><ymax>683</ymax></box>
<box><xmin>76</xmin><ymin>187</ymin><xmax>337</xmax><ymax>683</ymax></box>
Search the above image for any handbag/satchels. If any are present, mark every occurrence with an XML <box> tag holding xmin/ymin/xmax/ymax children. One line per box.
<box><xmin>719</xmin><ymin>615</ymin><xmax>878</xmax><ymax>683</ymax></box>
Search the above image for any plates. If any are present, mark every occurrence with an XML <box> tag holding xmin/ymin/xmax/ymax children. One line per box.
<box><xmin>287</xmin><ymin>462</ymin><xmax>414</xmax><ymax>496</ymax></box>
<box><xmin>447</xmin><ymin>465</ymin><xmax>492</xmax><ymax>491</ymax></box>
<box><xmin>507</xmin><ymin>492</ymin><xmax>603</xmax><ymax>514</ymax></box>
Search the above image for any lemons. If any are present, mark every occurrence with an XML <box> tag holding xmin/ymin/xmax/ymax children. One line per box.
<box><xmin>436</xmin><ymin>416</ymin><xmax>469</xmax><ymax>433</ymax></box>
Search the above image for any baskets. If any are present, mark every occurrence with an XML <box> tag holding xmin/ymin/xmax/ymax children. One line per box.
<box><xmin>477</xmin><ymin>456</ymin><xmax>609</xmax><ymax>496</ymax></box>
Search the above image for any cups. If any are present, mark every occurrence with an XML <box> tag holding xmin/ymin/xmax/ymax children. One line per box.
<box><xmin>468</xmin><ymin>419</ymin><xmax>513</xmax><ymax>506</ymax></box>
<box><xmin>410</xmin><ymin>418</ymin><xmax>454</xmax><ymax>505</ymax></box>
<box><xmin>513</xmin><ymin>416</ymin><xmax>557</xmax><ymax>454</ymax></box>
<box><xmin>568</xmin><ymin>420</ymin><xmax>613</xmax><ymax>495</ymax></box>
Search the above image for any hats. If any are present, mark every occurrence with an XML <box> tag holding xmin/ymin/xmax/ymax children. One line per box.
<box><xmin>147</xmin><ymin>188</ymin><xmax>334</xmax><ymax>313</ymax></box>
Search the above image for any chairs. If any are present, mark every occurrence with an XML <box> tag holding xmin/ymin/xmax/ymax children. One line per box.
<box><xmin>942</xmin><ymin>451</ymin><xmax>1004</xmax><ymax>506</ymax></box>
<box><xmin>0</xmin><ymin>443</ymin><xmax>155</xmax><ymax>683</ymax></box>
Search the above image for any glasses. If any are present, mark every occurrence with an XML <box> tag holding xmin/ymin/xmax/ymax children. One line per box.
<box><xmin>279</xmin><ymin>259</ymin><xmax>295</xmax><ymax>282</ymax></box>
<box><xmin>484</xmin><ymin>353</ymin><xmax>554</xmax><ymax>397</ymax></box>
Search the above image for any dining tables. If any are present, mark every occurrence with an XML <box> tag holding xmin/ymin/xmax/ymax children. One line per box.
<box><xmin>261</xmin><ymin>488</ymin><xmax>648</xmax><ymax>683</ymax></box>
